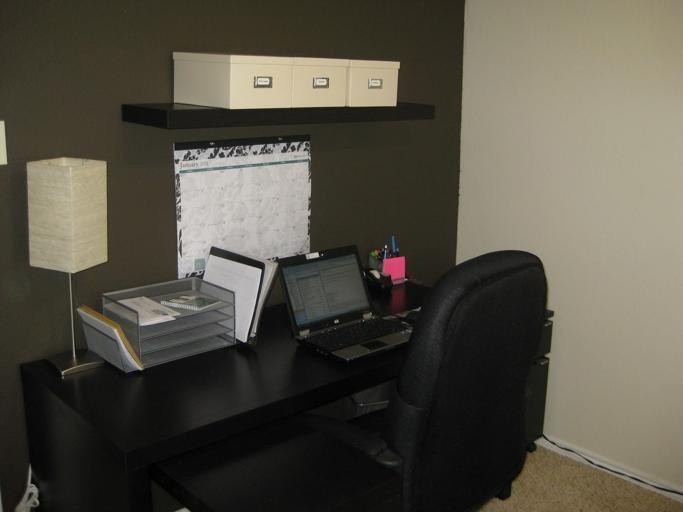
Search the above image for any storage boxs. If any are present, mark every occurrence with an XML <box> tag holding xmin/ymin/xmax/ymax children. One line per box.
<box><xmin>173</xmin><ymin>49</ymin><xmax>403</xmax><ymax>109</ymax></box>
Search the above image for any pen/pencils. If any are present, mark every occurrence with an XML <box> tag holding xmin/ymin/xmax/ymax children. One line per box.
<box><xmin>368</xmin><ymin>234</ymin><xmax>400</xmax><ymax>259</ymax></box>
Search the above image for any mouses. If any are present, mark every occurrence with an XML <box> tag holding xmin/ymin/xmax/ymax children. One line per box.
<box><xmin>406</xmin><ymin>311</ymin><xmax>419</xmax><ymax>320</ymax></box>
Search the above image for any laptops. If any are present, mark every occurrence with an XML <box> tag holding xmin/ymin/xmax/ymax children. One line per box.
<box><xmin>276</xmin><ymin>244</ymin><xmax>409</xmax><ymax>363</ymax></box>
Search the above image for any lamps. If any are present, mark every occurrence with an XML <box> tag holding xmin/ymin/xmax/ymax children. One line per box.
<box><xmin>25</xmin><ymin>155</ymin><xmax>113</xmax><ymax>383</ymax></box>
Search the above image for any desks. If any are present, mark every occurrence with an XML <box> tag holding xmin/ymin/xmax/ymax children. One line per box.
<box><xmin>19</xmin><ymin>278</ymin><xmax>555</xmax><ymax>511</ymax></box>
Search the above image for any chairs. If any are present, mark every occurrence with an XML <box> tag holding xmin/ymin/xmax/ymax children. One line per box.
<box><xmin>174</xmin><ymin>247</ymin><xmax>551</xmax><ymax>512</ymax></box>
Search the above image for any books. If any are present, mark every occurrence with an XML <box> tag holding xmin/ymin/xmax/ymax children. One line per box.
<box><xmin>160</xmin><ymin>294</ymin><xmax>219</xmax><ymax>311</ymax></box>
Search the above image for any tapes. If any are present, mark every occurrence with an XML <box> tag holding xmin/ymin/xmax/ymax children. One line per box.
<box><xmin>370</xmin><ymin>269</ymin><xmax>390</xmax><ymax>280</ymax></box>
